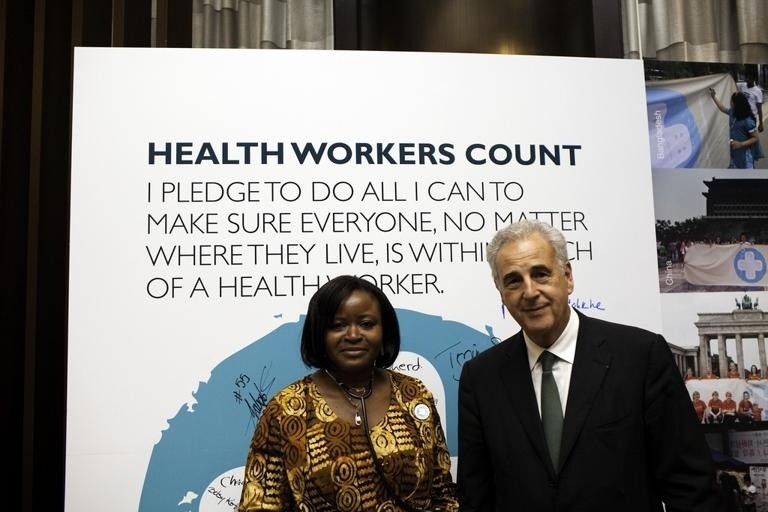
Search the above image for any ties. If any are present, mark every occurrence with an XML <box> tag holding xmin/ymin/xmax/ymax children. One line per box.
<box><xmin>534</xmin><ymin>349</ymin><xmax>566</xmax><ymax>479</ymax></box>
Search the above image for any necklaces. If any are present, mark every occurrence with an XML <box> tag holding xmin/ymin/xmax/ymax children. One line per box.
<box><xmin>324</xmin><ymin>368</ymin><xmax>376</xmax><ymax>426</ymax></box>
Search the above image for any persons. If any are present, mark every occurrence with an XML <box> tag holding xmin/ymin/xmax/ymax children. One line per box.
<box><xmin>746</xmin><ymin>364</ymin><xmax>761</xmax><ymax>383</ymax></box>
<box><xmin>721</xmin><ymin>391</ymin><xmax>737</xmax><ymax>424</ymax></box>
<box><xmin>236</xmin><ymin>274</ymin><xmax>460</xmax><ymax>512</ymax></box>
<box><xmin>748</xmin><ymin>80</ymin><xmax>765</xmax><ymax>133</ymax></box>
<box><xmin>707</xmin><ymin>390</ymin><xmax>723</xmax><ymax>425</ymax></box>
<box><xmin>692</xmin><ymin>390</ymin><xmax>707</xmax><ymax>424</ymax></box>
<box><xmin>737</xmin><ymin>391</ymin><xmax>753</xmax><ymax>422</ymax></box>
<box><xmin>700</xmin><ymin>366</ymin><xmax>719</xmax><ymax>379</ymax></box>
<box><xmin>458</xmin><ymin>219</ymin><xmax>719</xmax><ymax>511</ymax></box>
<box><xmin>684</xmin><ymin>366</ymin><xmax>700</xmax><ymax>382</ymax></box>
<box><xmin>726</xmin><ymin>362</ymin><xmax>741</xmax><ymax>379</ymax></box>
<box><xmin>710</xmin><ymin>88</ymin><xmax>760</xmax><ymax>169</ymax></box>
<box><xmin>657</xmin><ymin>233</ymin><xmax>752</xmax><ymax>269</ymax></box>
<box><xmin>751</xmin><ymin>404</ymin><xmax>764</xmax><ymax>421</ymax></box>
<box><xmin>717</xmin><ymin>471</ymin><xmax>757</xmax><ymax>512</ymax></box>
